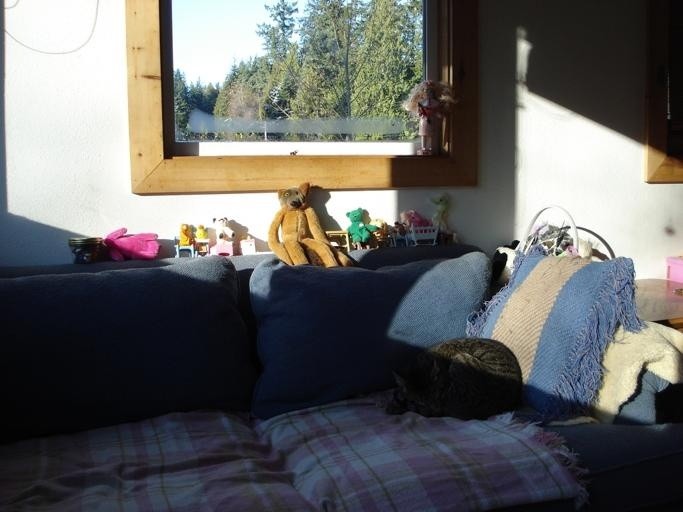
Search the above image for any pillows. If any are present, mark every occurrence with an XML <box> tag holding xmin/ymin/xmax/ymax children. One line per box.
<box><xmin>244</xmin><ymin>250</ymin><xmax>495</xmax><ymax>422</ymax></box>
<box><xmin>467</xmin><ymin>245</ymin><xmax>636</xmax><ymax>421</ymax></box>
<box><xmin>0</xmin><ymin>255</ymin><xmax>251</xmax><ymax>443</ymax></box>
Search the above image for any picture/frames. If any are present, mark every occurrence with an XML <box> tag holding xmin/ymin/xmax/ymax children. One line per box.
<box><xmin>324</xmin><ymin>230</ymin><xmax>350</xmax><ymax>253</ymax></box>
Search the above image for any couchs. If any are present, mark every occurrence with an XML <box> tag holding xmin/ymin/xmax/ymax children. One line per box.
<box><xmin>0</xmin><ymin>245</ymin><xmax>682</xmax><ymax>512</ymax></box>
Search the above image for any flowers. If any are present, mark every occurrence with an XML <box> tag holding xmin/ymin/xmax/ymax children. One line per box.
<box><xmin>399</xmin><ymin>81</ymin><xmax>457</xmax><ymax>128</ymax></box>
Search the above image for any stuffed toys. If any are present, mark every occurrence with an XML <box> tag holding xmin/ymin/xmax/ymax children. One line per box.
<box><xmin>368</xmin><ymin>206</ymin><xmax>432</xmax><ymax>248</ymax></box>
<box><xmin>266</xmin><ymin>180</ymin><xmax>354</xmax><ymax>268</ymax></box>
<box><xmin>178</xmin><ymin>223</ymin><xmax>193</xmax><ymax>247</ymax></box>
<box><xmin>211</xmin><ymin>216</ymin><xmax>235</xmax><ymax>242</ymax></box>
<box><xmin>400</xmin><ymin>79</ymin><xmax>459</xmax><ymax>159</ymax></box>
<box><xmin>427</xmin><ymin>191</ymin><xmax>452</xmax><ymax>232</ymax></box>
<box><xmin>194</xmin><ymin>224</ymin><xmax>208</xmax><ymax>241</ymax></box>
<box><xmin>70</xmin><ymin>226</ymin><xmax>162</xmax><ymax>262</ymax></box>
<box><xmin>343</xmin><ymin>207</ymin><xmax>375</xmax><ymax>251</ymax></box>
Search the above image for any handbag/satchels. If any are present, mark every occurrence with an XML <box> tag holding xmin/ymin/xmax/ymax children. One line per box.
<box><xmin>491</xmin><ymin>238</ymin><xmax>592</xmax><ymax>285</ymax></box>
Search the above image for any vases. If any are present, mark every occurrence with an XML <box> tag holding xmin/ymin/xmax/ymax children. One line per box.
<box><xmin>417</xmin><ymin>114</ymin><xmax>442</xmax><ymax>156</ymax></box>
